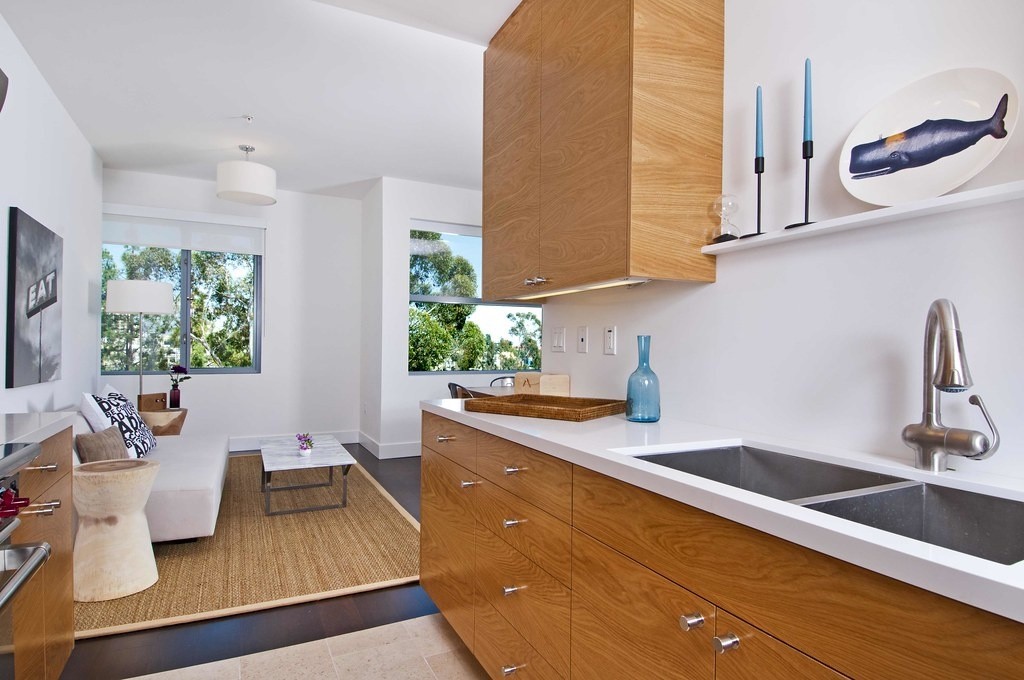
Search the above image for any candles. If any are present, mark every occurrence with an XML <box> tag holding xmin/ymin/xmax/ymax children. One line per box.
<box><xmin>756</xmin><ymin>59</ymin><xmax>812</xmax><ymax>157</ymax></box>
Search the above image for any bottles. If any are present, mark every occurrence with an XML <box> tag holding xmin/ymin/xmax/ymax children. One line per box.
<box><xmin>626</xmin><ymin>335</ymin><xmax>661</xmax><ymax>423</ymax></box>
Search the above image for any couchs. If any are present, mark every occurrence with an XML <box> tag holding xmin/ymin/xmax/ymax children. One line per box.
<box><xmin>60</xmin><ymin>407</ymin><xmax>229</xmax><ymax>542</ymax></box>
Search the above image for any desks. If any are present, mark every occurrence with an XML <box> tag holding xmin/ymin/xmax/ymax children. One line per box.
<box><xmin>138</xmin><ymin>408</ymin><xmax>187</xmax><ymax>435</ymax></box>
<box><xmin>73</xmin><ymin>459</ymin><xmax>160</xmax><ymax>602</ymax></box>
<box><xmin>465</xmin><ymin>386</ymin><xmax>514</xmax><ymax>398</ymax></box>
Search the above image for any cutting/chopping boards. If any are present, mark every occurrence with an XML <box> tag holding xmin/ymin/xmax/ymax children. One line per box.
<box><xmin>464</xmin><ymin>393</ymin><xmax>628</xmax><ymax>422</ymax></box>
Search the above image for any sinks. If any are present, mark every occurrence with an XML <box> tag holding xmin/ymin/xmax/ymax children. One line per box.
<box><xmin>787</xmin><ymin>480</ymin><xmax>1024</xmax><ymax>570</ymax></box>
<box><xmin>607</xmin><ymin>438</ymin><xmax>924</xmax><ymax>506</ymax></box>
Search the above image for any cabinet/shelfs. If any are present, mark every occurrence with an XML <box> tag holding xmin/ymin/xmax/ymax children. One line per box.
<box><xmin>11</xmin><ymin>426</ymin><xmax>74</xmax><ymax>680</ymax></box>
<box><xmin>420</xmin><ymin>410</ymin><xmax>1024</xmax><ymax>680</ymax></box>
<box><xmin>483</xmin><ymin>0</ymin><xmax>724</xmax><ymax>304</ymax></box>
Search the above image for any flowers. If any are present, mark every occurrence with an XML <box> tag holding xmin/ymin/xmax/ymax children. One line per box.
<box><xmin>296</xmin><ymin>433</ymin><xmax>314</xmax><ymax>450</ymax></box>
<box><xmin>168</xmin><ymin>365</ymin><xmax>191</xmax><ymax>397</ymax></box>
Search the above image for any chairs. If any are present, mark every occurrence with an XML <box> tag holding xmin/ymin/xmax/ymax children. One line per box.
<box><xmin>448</xmin><ymin>376</ymin><xmax>516</xmax><ymax>398</ymax></box>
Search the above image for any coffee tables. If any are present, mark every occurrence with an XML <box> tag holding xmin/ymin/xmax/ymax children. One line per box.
<box><xmin>259</xmin><ymin>435</ymin><xmax>357</xmax><ymax>516</ymax></box>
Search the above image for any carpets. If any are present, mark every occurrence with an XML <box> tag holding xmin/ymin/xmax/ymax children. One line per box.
<box><xmin>74</xmin><ymin>454</ymin><xmax>421</xmax><ymax>639</ymax></box>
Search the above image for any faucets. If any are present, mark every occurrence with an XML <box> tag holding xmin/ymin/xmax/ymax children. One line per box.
<box><xmin>899</xmin><ymin>296</ymin><xmax>1003</xmax><ymax>475</ymax></box>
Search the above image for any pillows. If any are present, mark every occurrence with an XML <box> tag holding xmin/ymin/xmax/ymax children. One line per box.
<box><xmin>75</xmin><ymin>384</ymin><xmax>158</xmax><ymax>463</ymax></box>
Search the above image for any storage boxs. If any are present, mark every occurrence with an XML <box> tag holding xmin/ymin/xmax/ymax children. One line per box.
<box><xmin>515</xmin><ymin>372</ymin><xmax>569</xmax><ymax>397</ymax></box>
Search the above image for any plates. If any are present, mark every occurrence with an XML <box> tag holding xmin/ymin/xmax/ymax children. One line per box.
<box><xmin>839</xmin><ymin>67</ymin><xmax>1020</xmax><ymax>207</ymax></box>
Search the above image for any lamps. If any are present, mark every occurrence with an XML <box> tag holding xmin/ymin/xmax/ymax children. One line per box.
<box><xmin>217</xmin><ymin>144</ymin><xmax>277</xmax><ymax>205</ymax></box>
<box><xmin>107</xmin><ymin>279</ymin><xmax>174</xmax><ymax>412</ymax></box>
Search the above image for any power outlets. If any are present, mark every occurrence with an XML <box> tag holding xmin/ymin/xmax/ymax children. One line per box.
<box><xmin>605</xmin><ymin>326</ymin><xmax>615</xmax><ymax>355</ymax></box>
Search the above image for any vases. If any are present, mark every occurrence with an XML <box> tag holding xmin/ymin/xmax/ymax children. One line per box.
<box><xmin>300</xmin><ymin>449</ymin><xmax>311</xmax><ymax>456</ymax></box>
<box><xmin>627</xmin><ymin>335</ymin><xmax>661</xmax><ymax>422</ymax></box>
<box><xmin>169</xmin><ymin>385</ymin><xmax>181</xmax><ymax>410</ymax></box>
